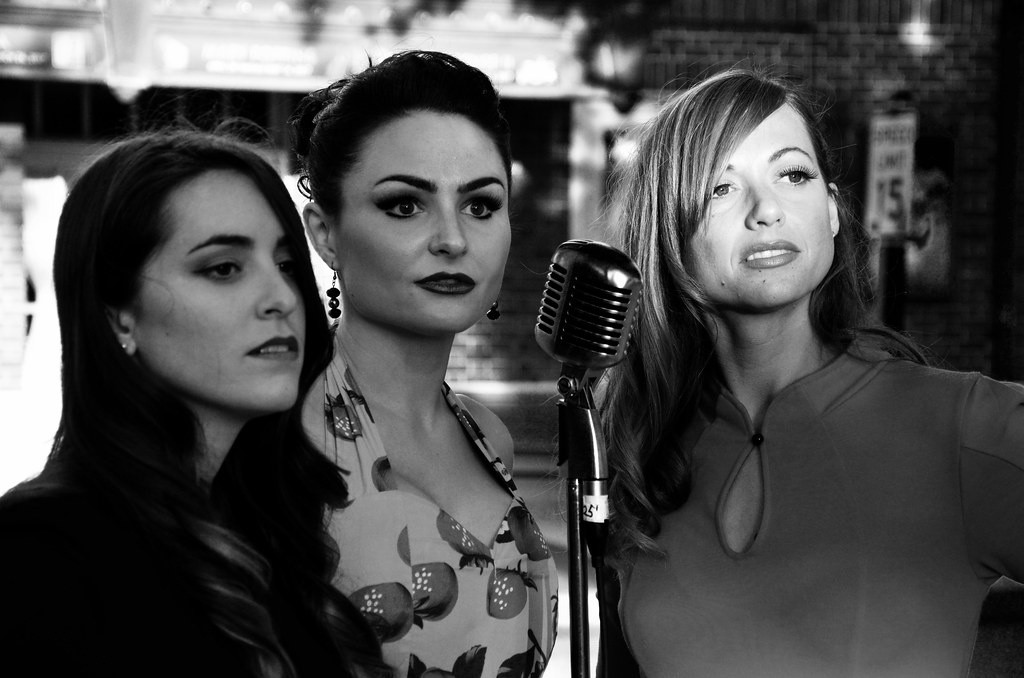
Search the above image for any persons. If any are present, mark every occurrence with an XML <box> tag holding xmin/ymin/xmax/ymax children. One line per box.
<box><xmin>0</xmin><ymin>133</ymin><xmax>399</xmax><ymax>678</ymax></box>
<box><xmin>599</xmin><ymin>73</ymin><xmax>1024</xmax><ymax>678</ymax></box>
<box><xmin>301</xmin><ymin>50</ymin><xmax>558</xmax><ymax>678</ymax></box>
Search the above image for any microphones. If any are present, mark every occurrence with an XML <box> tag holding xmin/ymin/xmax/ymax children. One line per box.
<box><xmin>534</xmin><ymin>240</ymin><xmax>644</xmax><ymax>568</ymax></box>
